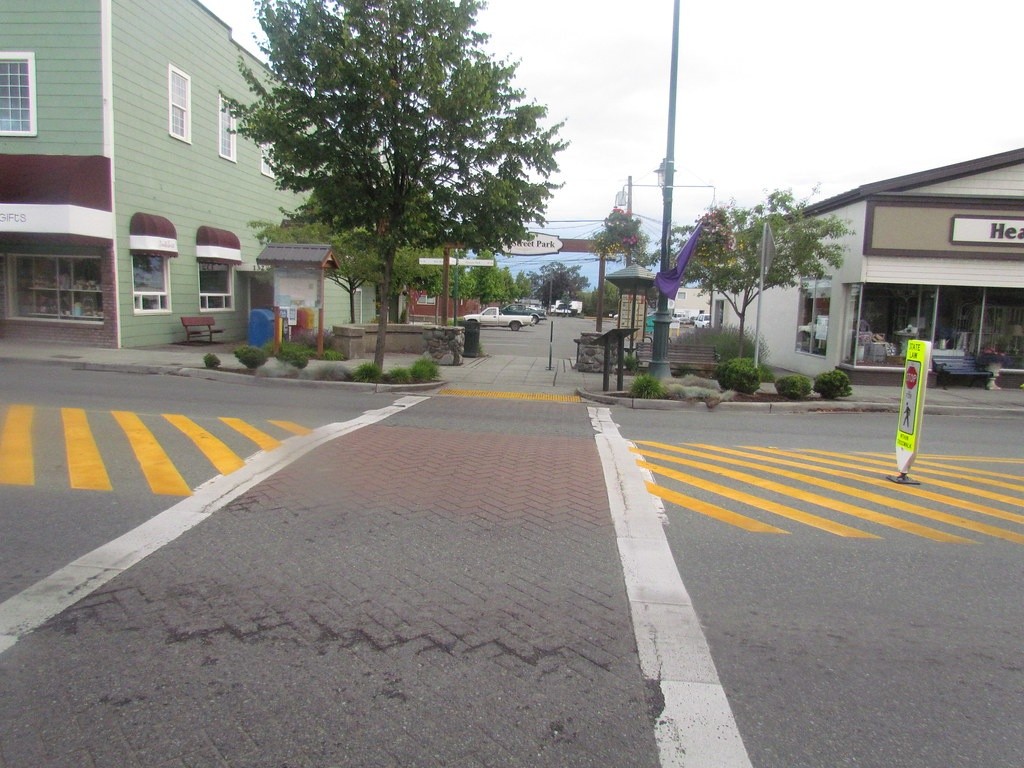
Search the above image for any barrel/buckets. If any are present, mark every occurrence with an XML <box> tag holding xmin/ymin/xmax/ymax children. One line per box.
<box><xmin>864</xmin><ymin>343</ymin><xmax>887</xmax><ymax>364</ymax></box>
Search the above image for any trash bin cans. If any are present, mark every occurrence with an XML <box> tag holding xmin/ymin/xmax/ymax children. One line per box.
<box><xmin>248</xmin><ymin>308</ymin><xmax>275</xmax><ymax>348</ymax></box>
<box><xmin>462</xmin><ymin>319</ymin><xmax>482</xmax><ymax>358</ymax></box>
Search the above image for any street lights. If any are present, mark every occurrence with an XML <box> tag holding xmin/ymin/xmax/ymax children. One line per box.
<box><xmin>616</xmin><ymin>175</ymin><xmax>635</xmax><ymax>267</ymax></box>
<box><xmin>648</xmin><ymin>158</ymin><xmax>673</xmax><ymax>386</ymax></box>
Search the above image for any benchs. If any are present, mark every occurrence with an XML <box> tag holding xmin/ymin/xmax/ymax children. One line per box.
<box><xmin>636</xmin><ymin>342</ymin><xmax>721</xmax><ymax>379</ymax></box>
<box><xmin>931</xmin><ymin>348</ymin><xmax>994</xmax><ymax>391</ymax></box>
<box><xmin>181</xmin><ymin>317</ymin><xmax>226</xmax><ymax>345</ymax></box>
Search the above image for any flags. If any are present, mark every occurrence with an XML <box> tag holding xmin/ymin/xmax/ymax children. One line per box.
<box><xmin>653</xmin><ymin>224</ymin><xmax>703</xmax><ymax>300</ymax></box>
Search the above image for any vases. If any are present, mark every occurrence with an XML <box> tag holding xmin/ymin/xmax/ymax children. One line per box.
<box><xmin>986</xmin><ymin>362</ymin><xmax>1001</xmax><ymax>390</ymax></box>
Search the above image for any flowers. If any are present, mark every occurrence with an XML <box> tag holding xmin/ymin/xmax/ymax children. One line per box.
<box><xmin>980</xmin><ymin>346</ymin><xmax>1006</xmax><ymax>365</ymax></box>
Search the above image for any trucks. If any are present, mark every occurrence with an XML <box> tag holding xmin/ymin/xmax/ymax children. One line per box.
<box><xmin>548</xmin><ymin>300</ymin><xmax>582</xmax><ymax>316</ymax></box>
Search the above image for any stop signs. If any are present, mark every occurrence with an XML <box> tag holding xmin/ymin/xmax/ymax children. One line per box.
<box><xmin>886</xmin><ymin>339</ymin><xmax>932</xmax><ymax>484</ymax></box>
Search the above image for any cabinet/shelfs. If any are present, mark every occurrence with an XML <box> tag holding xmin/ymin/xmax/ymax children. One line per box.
<box><xmin>27</xmin><ymin>275</ymin><xmax>103</xmax><ymax>321</ymax></box>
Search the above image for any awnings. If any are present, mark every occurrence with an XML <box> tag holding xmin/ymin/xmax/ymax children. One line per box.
<box><xmin>197</xmin><ymin>226</ymin><xmax>241</xmax><ymax>266</ymax></box>
<box><xmin>0</xmin><ymin>154</ymin><xmax>111</xmax><ymax>246</ymax></box>
<box><xmin>130</xmin><ymin>212</ymin><xmax>179</xmax><ymax>257</ymax></box>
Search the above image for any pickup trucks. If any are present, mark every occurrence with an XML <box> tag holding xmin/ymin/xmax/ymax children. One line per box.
<box><xmin>464</xmin><ymin>307</ymin><xmax>533</xmax><ymax>331</ymax></box>
<box><xmin>499</xmin><ymin>304</ymin><xmax>547</xmax><ymax>325</ymax></box>
